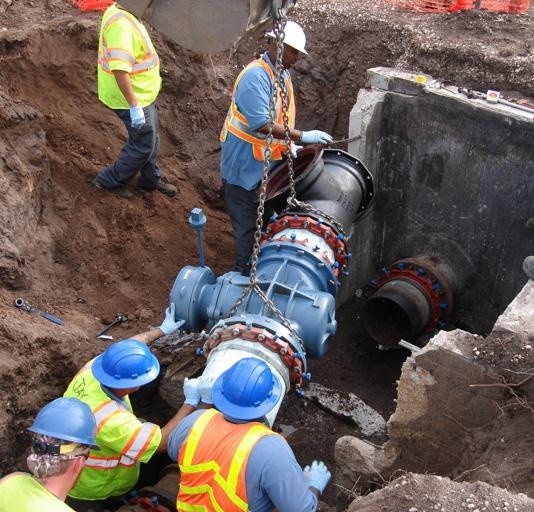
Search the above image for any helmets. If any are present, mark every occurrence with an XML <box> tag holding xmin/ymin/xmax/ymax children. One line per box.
<box><xmin>26</xmin><ymin>397</ymin><xmax>101</xmax><ymax>452</ymax></box>
<box><xmin>211</xmin><ymin>356</ymin><xmax>281</xmax><ymax>420</ymax></box>
<box><xmin>91</xmin><ymin>338</ymin><xmax>161</xmax><ymax>389</ymax></box>
<box><xmin>267</xmin><ymin>21</ymin><xmax>309</xmax><ymax>56</ymax></box>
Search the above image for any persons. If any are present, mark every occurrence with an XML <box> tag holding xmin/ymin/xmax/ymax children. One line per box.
<box><xmin>161</xmin><ymin>355</ymin><xmax>332</xmax><ymax>511</ymax></box>
<box><xmin>0</xmin><ymin>392</ymin><xmax>101</xmax><ymax>512</ymax></box>
<box><xmin>44</xmin><ymin>299</ymin><xmax>207</xmax><ymax>510</ymax></box>
<box><xmin>87</xmin><ymin>1</ymin><xmax>181</xmax><ymax>201</ymax></box>
<box><xmin>215</xmin><ymin>19</ymin><xmax>336</xmax><ymax>269</ymax></box>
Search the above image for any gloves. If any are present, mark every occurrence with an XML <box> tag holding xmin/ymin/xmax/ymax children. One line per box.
<box><xmin>303</xmin><ymin>461</ymin><xmax>331</xmax><ymax>495</ymax></box>
<box><xmin>159</xmin><ymin>303</ymin><xmax>186</xmax><ymax>336</ymax></box>
<box><xmin>182</xmin><ymin>376</ymin><xmax>214</xmax><ymax>408</ymax></box>
<box><xmin>291</xmin><ymin>143</ymin><xmax>303</xmax><ymax>158</ymax></box>
<box><xmin>301</xmin><ymin>130</ymin><xmax>335</xmax><ymax>144</ymax></box>
<box><xmin>128</xmin><ymin>106</ymin><xmax>146</xmax><ymax>129</ymax></box>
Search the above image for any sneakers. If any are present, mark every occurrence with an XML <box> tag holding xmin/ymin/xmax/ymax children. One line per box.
<box><xmin>93</xmin><ymin>178</ymin><xmax>134</xmax><ymax>198</ymax></box>
<box><xmin>140</xmin><ymin>180</ymin><xmax>177</xmax><ymax>197</ymax></box>
